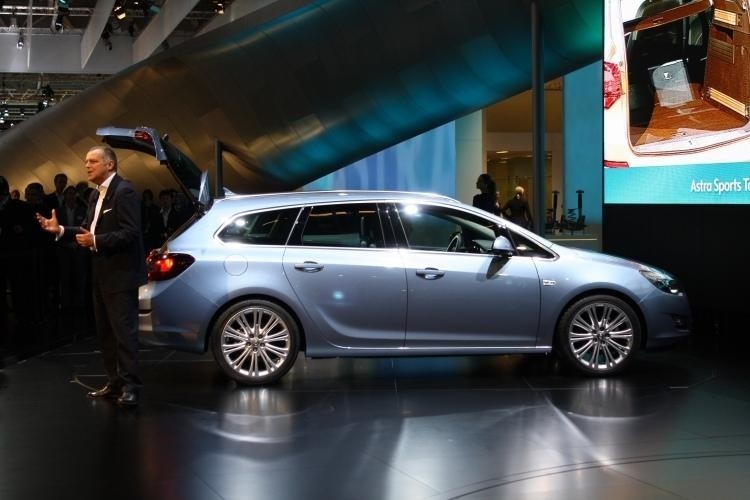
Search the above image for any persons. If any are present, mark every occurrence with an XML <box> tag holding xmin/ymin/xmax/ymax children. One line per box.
<box><xmin>33</xmin><ymin>139</ymin><xmax>148</xmax><ymax>408</ymax></box>
<box><xmin>501</xmin><ymin>185</ymin><xmax>532</xmax><ymax>228</ymax></box>
<box><xmin>1</xmin><ymin>169</ymin><xmax>193</xmax><ymax>364</ymax></box>
<box><xmin>495</xmin><ymin>190</ymin><xmax>503</xmax><ymax>219</ymax></box>
<box><xmin>473</xmin><ymin>172</ymin><xmax>498</xmax><ymax>215</ymax></box>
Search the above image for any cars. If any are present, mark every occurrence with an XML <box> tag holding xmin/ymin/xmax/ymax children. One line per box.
<box><xmin>96</xmin><ymin>126</ymin><xmax>691</xmax><ymax>387</ymax></box>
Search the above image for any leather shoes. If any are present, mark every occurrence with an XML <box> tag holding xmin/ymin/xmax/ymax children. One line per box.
<box><xmin>87</xmin><ymin>386</ymin><xmax>138</xmax><ymax>406</ymax></box>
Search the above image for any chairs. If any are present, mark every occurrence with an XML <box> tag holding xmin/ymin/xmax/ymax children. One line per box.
<box><xmin>359</xmin><ymin>213</ymin><xmax>413</xmax><ymax>250</ymax></box>
